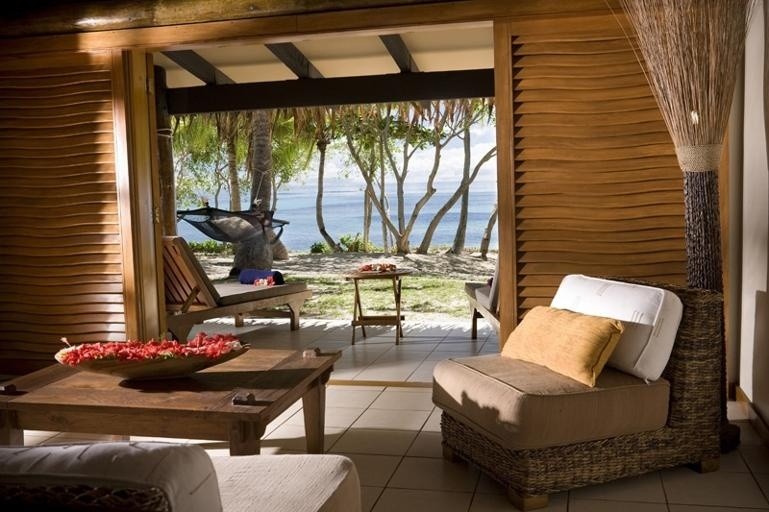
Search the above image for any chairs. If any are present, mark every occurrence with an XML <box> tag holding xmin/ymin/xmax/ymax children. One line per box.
<box><xmin>465</xmin><ymin>253</ymin><xmax>501</xmax><ymax>350</ymax></box>
<box><xmin>0</xmin><ymin>440</ymin><xmax>361</xmax><ymax>512</ymax></box>
<box><xmin>163</xmin><ymin>235</ymin><xmax>313</xmax><ymax>344</ymax></box>
<box><xmin>433</xmin><ymin>273</ymin><xmax>723</xmax><ymax>512</ymax></box>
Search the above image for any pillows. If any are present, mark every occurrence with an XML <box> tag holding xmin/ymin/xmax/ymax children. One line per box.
<box><xmin>501</xmin><ymin>305</ymin><xmax>624</xmax><ymax>387</ymax></box>
<box><xmin>549</xmin><ymin>274</ymin><xmax>684</xmax><ymax>382</ymax></box>
<box><xmin>213</xmin><ymin>217</ymin><xmax>257</xmax><ymax>243</ymax></box>
<box><xmin>239</xmin><ymin>268</ymin><xmax>284</xmax><ymax>287</ymax></box>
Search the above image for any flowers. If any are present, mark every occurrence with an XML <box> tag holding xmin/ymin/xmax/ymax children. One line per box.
<box><xmin>57</xmin><ymin>331</ymin><xmax>245</xmax><ymax>368</ymax></box>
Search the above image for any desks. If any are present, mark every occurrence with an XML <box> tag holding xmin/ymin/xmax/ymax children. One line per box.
<box><xmin>342</xmin><ymin>269</ymin><xmax>413</xmax><ymax>345</ymax></box>
